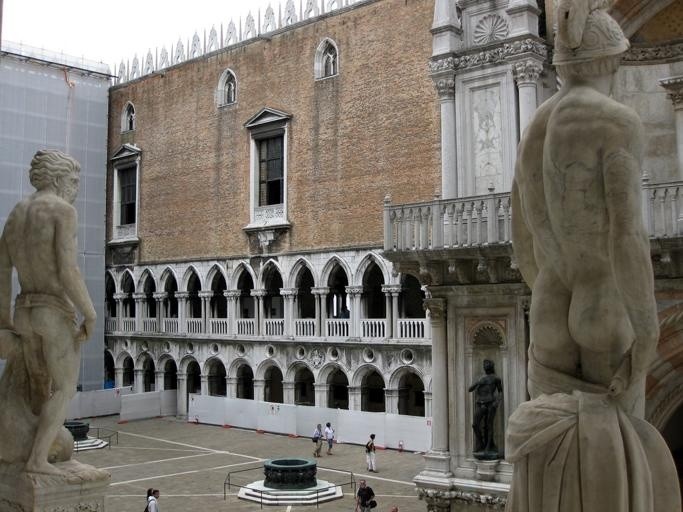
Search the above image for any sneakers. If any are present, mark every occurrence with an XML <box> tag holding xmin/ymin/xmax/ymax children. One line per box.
<box><xmin>369</xmin><ymin>469</ymin><xmax>378</xmax><ymax>473</ymax></box>
<box><xmin>313</xmin><ymin>452</ymin><xmax>322</xmax><ymax>457</ymax></box>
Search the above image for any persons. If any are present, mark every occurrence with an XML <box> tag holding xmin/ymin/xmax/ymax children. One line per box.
<box><xmin>508</xmin><ymin>8</ymin><xmax>661</xmax><ymax>426</ymax></box>
<box><xmin>324</xmin><ymin>421</ymin><xmax>335</xmax><ymax>455</ymax></box>
<box><xmin>146</xmin><ymin>487</ymin><xmax>153</xmax><ymax>497</ymax></box>
<box><xmin>0</xmin><ymin>149</ymin><xmax>96</xmax><ymax>475</ymax></box>
<box><xmin>390</xmin><ymin>504</ymin><xmax>398</xmax><ymax>512</ymax></box>
<box><xmin>354</xmin><ymin>479</ymin><xmax>374</xmax><ymax>511</ymax></box>
<box><xmin>311</xmin><ymin>423</ymin><xmax>324</xmax><ymax>457</ymax></box>
<box><xmin>365</xmin><ymin>433</ymin><xmax>378</xmax><ymax>473</ymax></box>
<box><xmin>468</xmin><ymin>358</ymin><xmax>504</xmax><ymax>454</ymax></box>
<box><xmin>145</xmin><ymin>489</ymin><xmax>160</xmax><ymax>512</ymax></box>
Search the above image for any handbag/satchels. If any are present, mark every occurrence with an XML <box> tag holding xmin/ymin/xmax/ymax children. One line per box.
<box><xmin>366</xmin><ymin>442</ymin><xmax>372</xmax><ymax>449</ymax></box>
<box><xmin>312</xmin><ymin>437</ymin><xmax>319</xmax><ymax>442</ymax></box>
